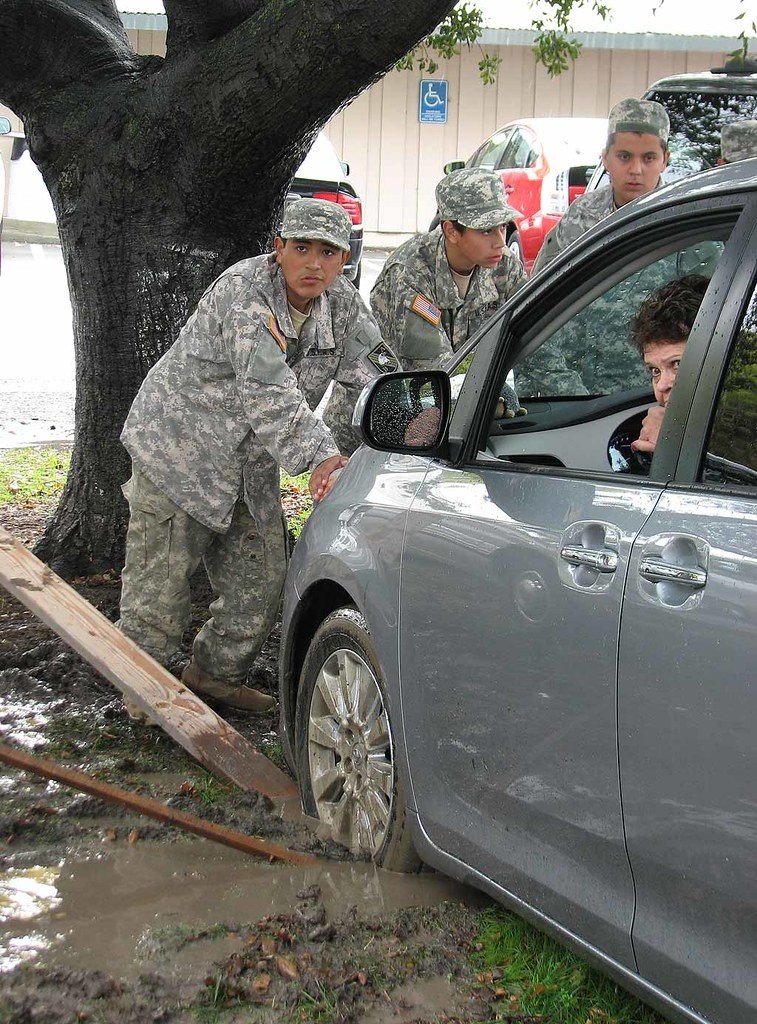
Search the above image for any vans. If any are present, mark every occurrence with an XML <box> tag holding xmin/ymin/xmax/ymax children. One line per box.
<box><xmin>584</xmin><ymin>58</ymin><xmax>756</xmax><ymax>194</ymax></box>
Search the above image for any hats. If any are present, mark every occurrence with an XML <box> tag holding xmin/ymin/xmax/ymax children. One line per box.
<box><xmin>720</xmin><ymin>119</ymin><xmax>757</xmax><ymax>162</ymax></box>
<box><xmin>281</xmin><ymin>198</ymin><xmax>352</xmax><ymax>252</ymax></box>
<box><xmin>435</xmin><ymin>168</ymin><xmax>524</xmax><ymax>232</ymax></box>
<box><xmin>607</xmin><ymin>97</ymin><xmax>670</xmax><ymax>144</ymax></box>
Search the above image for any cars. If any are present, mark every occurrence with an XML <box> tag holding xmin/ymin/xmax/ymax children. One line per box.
<box><xmin>428</xmin><ymin>118</ymin><xmax>610</xmax><ymax>280</ymax></box>
<box><xmin>280</xmin><ymin>157</ymin><xmax>756</xmax><ymax>1017</ymax></box>
<box><xmin>278</xmin><ymin>133</ymin><xmax>363</xmax><ymax>289</ymax></box>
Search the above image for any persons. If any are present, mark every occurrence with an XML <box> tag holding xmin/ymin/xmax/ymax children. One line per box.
<box><xmin>630</xmin><ymin>270</ymin><xmax>712</xmax><ymax>458</ymax></box>
<box><xmin>113</xmin><ymin>197</ymin><xmax>413</xmax><ymax>721</ymax></box>
<box><xmin>369</xmin><ymin>399</ymin><xmax>442</xmax><ymax>448</ymax></box>
<box><xmin>531</xmin><ymin>97</ymin><xmax>725</xmax><ymax>396</ymax></box>
<box><xmin>716</xmin><ymin>117</ymin><xmax>757</xmax><ymax>166</ymax></box>
<box><xmin>366</xmin><ymin>165</ymin><xmax>591</xmax><ymax>396</ymax></box>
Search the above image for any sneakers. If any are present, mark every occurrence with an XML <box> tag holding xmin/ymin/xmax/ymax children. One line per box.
<box><xmin>181</xmin><ymin>655</ymin><xmax>280</xmax><ymax>717</ymax></box>
<box><xmin>121</xmin><ymin>693</ymin><xmax>158</xmax><ymax>726</ymax></box>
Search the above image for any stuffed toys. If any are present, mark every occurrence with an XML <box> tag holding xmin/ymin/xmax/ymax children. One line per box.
<box><xmin>491</xmin><ymin>383</ymin><xmax>528</xmax><ymax>419</ymax></box>
